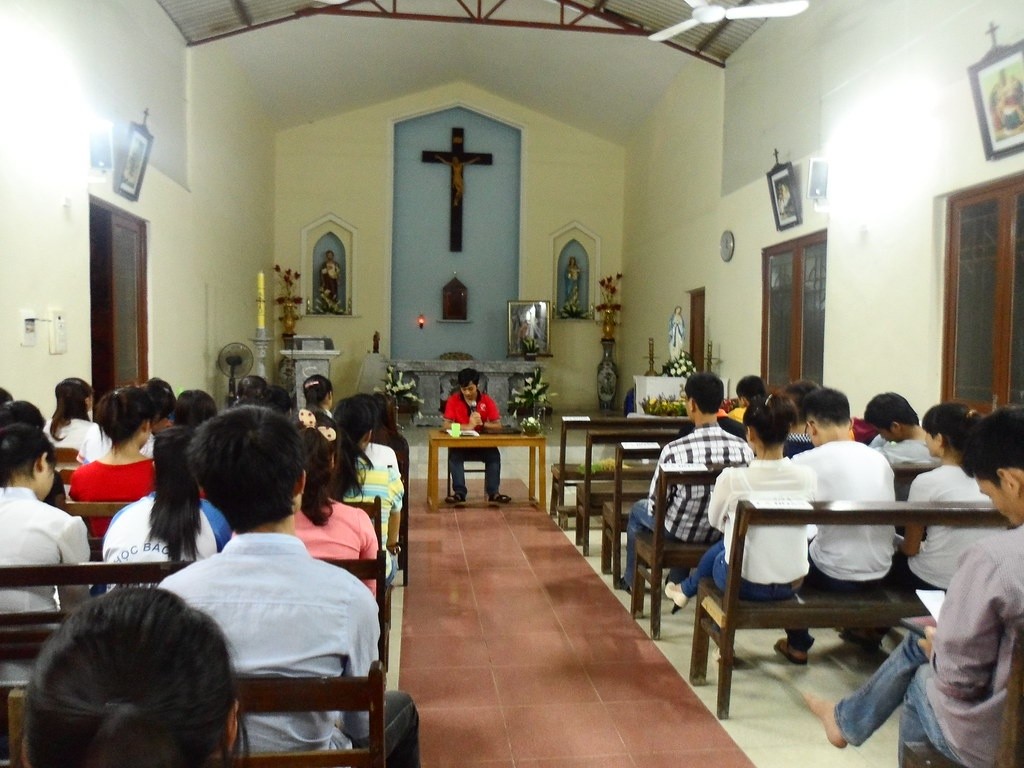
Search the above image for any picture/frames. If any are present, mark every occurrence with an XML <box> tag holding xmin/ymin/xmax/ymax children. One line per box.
<box><xmin>508</xmin><ymin>300</ymin><xmax>553</xmax><ymax>356</ymax></box>
<box><xmin>766</xmin><ymin>161</ymin><xmax>802</xmax><ymax>232</ymax></box>
<box><xmin>114</xmin><ymin>120</ymin><xmax>154</xmax><ymax>203</ymax></box>
<box><xmin>968</xmin><ymin>37</ymin><xmax>1024</xmax><ymax>162</ymax></box>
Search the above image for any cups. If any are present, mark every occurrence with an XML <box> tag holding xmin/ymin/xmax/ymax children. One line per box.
<box><xmin>451</xmin><ymin>423</ymin><xmax>460</xmax><ymax>438</ymax></box>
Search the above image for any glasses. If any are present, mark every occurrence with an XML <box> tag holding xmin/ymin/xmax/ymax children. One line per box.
<box><xmin>805</xmin><ymin>421</ymin><xmax>814</xmax><ymax>437</ymax></box>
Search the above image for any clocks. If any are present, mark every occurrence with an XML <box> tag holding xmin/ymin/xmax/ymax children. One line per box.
<box><xmin>720</xmin><ymin>230</ymin><xmax>734</xmax><ymax>262</ymax></box>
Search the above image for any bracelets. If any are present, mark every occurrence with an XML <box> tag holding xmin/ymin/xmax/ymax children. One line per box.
<box><xmin>482</xmin><ymin>422</ymin><xmax>484</xmax><ymax>428</ymax></box>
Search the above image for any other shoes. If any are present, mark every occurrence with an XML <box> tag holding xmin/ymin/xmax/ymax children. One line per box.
<box><xmin>665</xmin><ymin>574</ymin><xmax>669</xmax><ymax>585</ymax></box>
<box><xmin>839</xmin><ymin>630</ymin><xmax>883</xmax><ymax>649</ymax></box>
<box><xmin>776</xmin><ymin>638</ymin><xmax>807</xmax><ymax>664</ymax></box>
<box><xmin>620</xmin><ymin>577</ymin><xmax>631</xmax><ymax>594</ymax></box>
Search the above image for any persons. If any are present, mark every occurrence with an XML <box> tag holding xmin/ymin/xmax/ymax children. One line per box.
<box><xmin>442</xmin><ymin>368</ymin><xmax>512</xmax><ymax>503</ymax></box>
<box><xmin>617</xmin><ymin>372</ymin><xmax>1024</xmax><ymax>768</ymax></box>
<box><xmin>156</xmin><ymin>403</ymin><xmax>420</xmax><ymax>768</ymax></box>
<box><xmin>773</xmin><ymin>386</ymin><xmax>895</xmax><ymax>666</ymax></box>
<box><xmin>664</xmin><ymin>391</ymin><xmax>799</xmax><ymax>615</ymax></box>
<box><xmin>21</xmin><ymin>586</ymin><xmax>238</xmax><ymax>768</ymax></box>
<box><xmin>317</xmin><ymin>250</ymin><xmax>344</xmax><ymax>314</ymax></box>
<box><xmin>434</xmin><ymin>154</ymin><xmax>480</xmax><ymax>207</ymax></box>
<box><xmin>0</xmin><ymin>374</ymin><xmax>406</xmax><ymax>768</ymax></box>
<box><xmin>563</xmin><ymin>255</ymin><xmax>580</xmax><ymax>301</ymax></box>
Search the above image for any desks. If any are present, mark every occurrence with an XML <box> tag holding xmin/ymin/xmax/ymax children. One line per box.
<box><xmin>426</xmin><ymin>427</ymin><xmax>546</xmax><ymax>513</ymax></box>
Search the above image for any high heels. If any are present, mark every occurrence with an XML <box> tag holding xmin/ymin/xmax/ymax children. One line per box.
<box><xmin>665</xmin><ymin>582</ymin><xmax>690</xmax><ymax>614</ymax></box>
<box><xmin>713</xmin><ymin>646</ymin><xmax>735</xmax><ymax>665</ymax></box>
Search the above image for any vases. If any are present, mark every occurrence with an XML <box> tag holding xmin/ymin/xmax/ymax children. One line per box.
<box><xmin>597</xmin><ymin>338</ymin><xmax>617</xmax><ymax>411</ymax></box>
<box><xmin>280</xmin><ymin>332</ymin><xmax>297</xmax><ymax>395</ymax></box>
<box><xmin>515</xmin><ymin>407</ymin><xmax>533</xmax><ymax>420</ymax></box>
<box><xmin>395</xmin><ymin>401</ymin><xmax>417</xmax><ymax>420</ymax></box>
<box><xmin>603</xmin><ymin>308</ymin><xmax>616</xmax><ymax>339</ymax></box>
<box><xmin>279</xmin><ymin>303</ymin><xmax>298</xmax><ymax>332</ymax></box>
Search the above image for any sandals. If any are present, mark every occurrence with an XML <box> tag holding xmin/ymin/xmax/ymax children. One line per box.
<box><xmin>489</xmin><ymin>492</ymin><xmax>511</xmax><ymax>503</ymax></box>
<box><xmin>445</xmin><ymin>493</ymin><xmax>466</xmax><ymax>503</ymax></box>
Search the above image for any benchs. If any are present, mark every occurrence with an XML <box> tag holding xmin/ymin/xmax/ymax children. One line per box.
<box><xmin>0</xmin><ymin>417</ymin><xmax>410</xmax><ymax>768</ymax></box>
<box><xmin>545</xmin><ymin>411</ymin><xmax>1024</xmax><ymax>768</ymax></box>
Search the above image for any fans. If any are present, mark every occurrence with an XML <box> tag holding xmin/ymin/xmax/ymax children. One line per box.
<box><xmin>647</xmin><ymin>0</ymin><xmax>809</xmax><ymax>43</ymax></box>
<box><xmin>217</xmin><ymin>342</ymin><xmax>254</xmax><ymax>407</ymax></box>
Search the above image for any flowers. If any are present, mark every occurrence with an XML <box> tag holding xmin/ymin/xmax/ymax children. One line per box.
<box><xmin>597</xmin><ymin>272</ymin><xmax>623</xmax><ymax>310</ymax></box>
<box><xmin>641</xmin><ymin>394</ymin><xmax>737</xmax><ymax>417</ymax></box>
<box><xmin>506</xmin><ymin>366</ymin><xmax>559</xmax><ymax>432</ymax></box>
<box><xmin>664</xmin><ymin>352</ymin><xmax>697</xmax><ymax>378</ymax></box>
<box><xmin>272</xmin><ymin>263</ymin><xmax>304</xmax><ymax>305</ymax></box>
<box><xmin>382</xmin><ymin>365</ymin><xmax>425</xmax><ymax>417</ymax></box>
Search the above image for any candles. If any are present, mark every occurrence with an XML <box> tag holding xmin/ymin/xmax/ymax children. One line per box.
<box><xmin>256</xmin><ymin>271</ymin><xmax>265</xmax><ymax>328</ymax></box>
